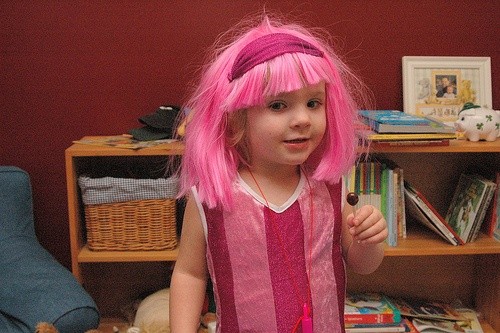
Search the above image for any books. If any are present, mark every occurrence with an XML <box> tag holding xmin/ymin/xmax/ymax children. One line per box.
<box><xmin>342</xmin><ymin>287</ymin><xmax>484</xmax><ymax>333</ymax></box>
<box><xmin>341</xmin><ymin>153</ymin><xmax>500</xmax><ymax>247</ymax></box>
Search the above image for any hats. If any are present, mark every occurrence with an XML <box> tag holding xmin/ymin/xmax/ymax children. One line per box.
<box><xmin>139</xmin><ymin>105</ymin><xmax>182</xmax><ymax>128</ymax></box>
<box><xmin>127</xmin><ymin>123</ymin><xmax>175</xmax><ymax>141</ymax></box>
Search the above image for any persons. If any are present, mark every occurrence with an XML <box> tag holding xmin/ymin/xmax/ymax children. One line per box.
<box><xmin>437</xmin><ymin>76</ymin><xmax>456</xmax><ymax>99</ymax></box>
<box><xmin>170</xmin><ymin>15</ymin><xmax>389</xmax><ymax>333</ymax></box>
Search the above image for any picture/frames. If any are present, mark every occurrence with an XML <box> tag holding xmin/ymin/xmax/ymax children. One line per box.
<box><xmin>401</xmin><ymin>56</ymin><xmax>492</xmax><ymax>126</ymax></box>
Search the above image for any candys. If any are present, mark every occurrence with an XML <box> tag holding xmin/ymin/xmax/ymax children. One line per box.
<box><xmin>346</xmin><ymin>192</ymin><xmax>359</xmax><ymax>217</ymax></box>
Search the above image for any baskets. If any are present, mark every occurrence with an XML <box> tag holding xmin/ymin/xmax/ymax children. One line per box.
<box><xmin>79</xmin><ymin>172</ymin><xmax>177</xmax><ymax>251</ymax></box>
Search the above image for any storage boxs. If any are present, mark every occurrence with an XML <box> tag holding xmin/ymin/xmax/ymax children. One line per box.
<box><xmin>76</xmin><ymin>172</ymin><xmax>179</xmax><ymax>250</ymax></box>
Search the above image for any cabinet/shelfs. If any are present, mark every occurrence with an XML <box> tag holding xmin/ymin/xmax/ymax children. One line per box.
<box><xmin>65</xmin><ymin>135</ymin><xmax>500</xmax><ymax>333</ymax></box>
